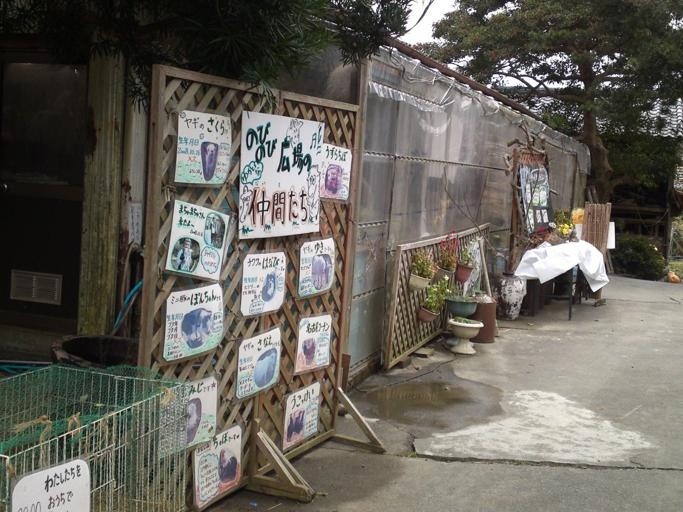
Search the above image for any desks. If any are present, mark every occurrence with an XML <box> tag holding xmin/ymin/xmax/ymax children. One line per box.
<box><xmin>528</xmin><ymin>240</ymin><xmax>589</xmax><ymax>321</ymax></box>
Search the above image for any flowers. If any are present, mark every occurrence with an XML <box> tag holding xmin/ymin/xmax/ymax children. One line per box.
<box><xmin>420</xmin><ymin>274</ymin><xmax>452</xmax><ymax>314</ymax></box>
<box><xmin>436</xmin><ymin>230</ymin><xmax>460</xmax><ymax>272</ymax></box>
<box><xmin>410</xmin><ymin>246</ymin><xmax>438</xmax><ymax>280</ymax></box>
<box><xmin>557</xmin><ymin>223</ymin><xmax>573</xmax><ymax>237</ymax></box>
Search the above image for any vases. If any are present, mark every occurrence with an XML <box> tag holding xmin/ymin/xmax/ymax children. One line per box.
<box><xmin>417</xmin><ymin>305</ymin><xmax>438</xmax><ymax>323</ymax></box>
<box><xmin>433</xmin><ymin>264</ymin><xmax>455</xmax><ymax>282</ymax></box>
<box><xmin>408</xmin><ymin>273</ymin><xmax>431</xmax><ymax>290</ymax></box>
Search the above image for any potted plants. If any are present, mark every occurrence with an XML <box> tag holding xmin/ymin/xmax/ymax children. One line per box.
<box><xmin>443</xmin><ymin>280</ymin><xmax>484</xmax><ymax>356</ymax></box>
<box><xmin>453</xmin><ymin>238</ymin><xmax>479</xmax><ymax>282</ymax></box>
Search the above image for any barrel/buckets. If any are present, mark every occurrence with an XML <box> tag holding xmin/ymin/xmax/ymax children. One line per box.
<box><xmin>50</xmin><ymin>335</ymin><xmax>138</xmax><ymax>441</ymax></box>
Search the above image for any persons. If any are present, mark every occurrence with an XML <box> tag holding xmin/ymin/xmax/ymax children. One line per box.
<box><xmin>209</xmin><ymin>215</ymin><xmax>217</xmax><ymax>245</ymax></box>
<box><xmin>176</xmin><ymin>239</ymin><xmax>194</xmax><ymax>272</ymax></box>
